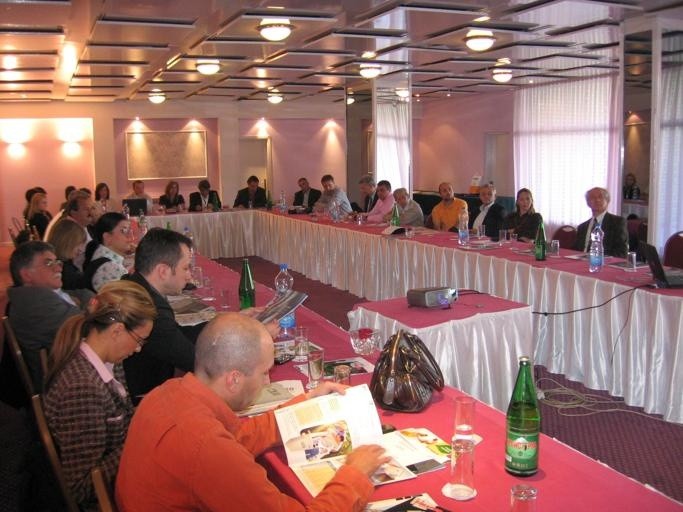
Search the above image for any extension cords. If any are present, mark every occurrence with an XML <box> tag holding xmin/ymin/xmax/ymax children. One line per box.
<box><xmin>535</xmin><ymin>387</ymin><xmax>545</xmax><ymax>400</ymax></box>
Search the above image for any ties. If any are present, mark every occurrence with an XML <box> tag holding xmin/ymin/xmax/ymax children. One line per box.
<box><xmin>586</xmin><ymin>218</ymin><xmax>597</xmax><ymax>253</ymax></box>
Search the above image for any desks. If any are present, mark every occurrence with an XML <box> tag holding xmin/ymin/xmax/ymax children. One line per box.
<box><xmin>47</xmin><ymin>206</ymin><xmax>683</xmax><ymax>512</ymax></box>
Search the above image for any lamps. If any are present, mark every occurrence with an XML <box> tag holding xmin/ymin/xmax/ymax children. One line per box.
<box><xmin>254</xmin><ymin>17</ymin><xmax>296</xmax><ymax>42</ymax></box>
<box><xmin>358</xmin><ymin>64</ymin><xmax>382</xmax><ymax>79</ymax></box>
<box><xmin>265</xmin><ymin>94</ymin><xmax>285</xmax><ymax>104</ymax></box>
<box><xmin>490</xmin><ymin>68</ymin><xmax>515</xmax><ymax>84</ymax></box>
<box><xmin>195</xmin><ymin>59</ymin><xmax>222</xmax><ymax>75</ymax></box>
<box><xmin>460</xmin><ymin>28</ymin><xmax>497</xmax><ymax>52</ymax></box>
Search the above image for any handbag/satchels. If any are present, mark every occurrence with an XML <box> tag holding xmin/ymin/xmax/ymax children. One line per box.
<box><xmin>369</xmin><ymin>329</ymin><xmax>444</xmax><ymax>413</ymax></box>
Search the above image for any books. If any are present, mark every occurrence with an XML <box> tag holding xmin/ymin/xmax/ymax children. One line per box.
<box><xmin>564</xmin><ymin>252</ymin><xmax>613</xmax><ymax>261</ymax></box>
<box><xmin>608</xmin><ymin>261</ymin><xmax>650</xmax><ymax>270</ymax></box>
<box><xmin>234</xmin><ymin>380</ymin><xmax>304</xmax><ymax>417</ymax></box>
<box><xmin>253</xmin><ymin>289</ymin><xmax>307</xmax><ymax>323</ymax></box>
<box><xmin>166</xmin><ymin>293</ymin><xmax>219</xmax><ymax>327</ymax></box>
<box><xmin>273</xmin><ymin>383</ymin><xmax>447</xmax><ymax>499</ymax></box>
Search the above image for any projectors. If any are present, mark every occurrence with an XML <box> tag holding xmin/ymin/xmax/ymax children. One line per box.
<box><xmin>407</xmin><ymin>287</ymin><xmax>458</xmax><ymax>308</ymax></box>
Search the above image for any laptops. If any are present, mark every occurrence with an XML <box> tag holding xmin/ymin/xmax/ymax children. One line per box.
<box><xmin>123</xmin><ymin>199</ymin><xmax>148</xmax><ymax>216</ymax></box>
<box><xmin>639</xmin><ymin>240</ymin><xmax>683</xmax><ymax>287</ymax></box>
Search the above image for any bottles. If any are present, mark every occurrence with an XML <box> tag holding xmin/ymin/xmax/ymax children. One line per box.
<box><xmin>505</xmin><ymin>357</ymin><xmax>541</xmax><ymax>476</ymax></box>
<box><xmin>182</xmin><ymin>227</ymin><xmax>195</xmax><ymax>271</ymax></box>
<box><xmin>239</xmin><ymin>257</ymin><xmax>255</xmax><ymax>310</ymax></box>
<box><xmin>165</xmin><ymin>222</ymin><xmax>172</xmax><ymax>232</ymax></box>
<box><xmin>458</xmin><ymin>206</ymin><xmax>470</xmax><ymax>246</ymax></box>
<box><xmin>122</xmin><ymin>204</ymin><xmax>130</xmax><ymax>217</ymax></box>
<box><xmin>534</xmin><ymin>220</ymin><xmax>546</xmax><ymax>260</ymax></box>
<box><xmin>329</xmin><ymin>194</ymin><xmax>338</xmax><ymax>219</ymax></box>
<box><xmin>279</xmin><ymin>190</ymin><xmax>286</xmax><ymax>211</ymax></box>
<box><xmin>551</xmin><ymin>240</ymin><xmax>560</xmax><ymax>257</ymax></box>
<box><xmin>392</xmin><ymin>202</ymin><xmax>400</xmax><ymax>226</ymax></box>
<box><xmin>137</xmin><ymin>212</ymin><xmax>148</xmax><ymax>234</ymax></box>
<box><xmin>275</xmin><ymin>263</ymin><xmax>295</xmax><ymax>329</ymax></box>
<box><xmin>125</xmin><ymin>212</ymin><xmax>132</xmax><ymax>228</ymax></box>
<box><xmin>589</xmin><ymin>224</ymin><xmax>604</xmax><ymax>275</ymax></box>
<box><xmin>627</xmin><ymin>253</ymin><xmax>636</xmax><ymax>271</ymax></box>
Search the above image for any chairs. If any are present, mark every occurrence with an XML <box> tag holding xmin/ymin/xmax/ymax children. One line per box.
<box><xmin>32</xmin><ymin>395</ymin><xmax>73</xmax><ymax>510</ymax></box>
<box><xmin>8</xmin><ymin>227</ymin><xmax>16</xmax><ymax>248</ymax></box>
<box><xmin>551</xmin><ymin>225</ymin><xmax>577</xmax><ymax>250</ymax></box>
<box><xmin>625</xmin><ymin>210</ymin><xmax>649</xmax><ymax>258</ymax></box>
<box><xmin>2</xmin><ymin>316</ymin><xmax>34</xmax><ymax>395</ymax></box>
<box><xmin>662</xmin><ymin>229</ymin><xmax>683</xmax><ymax>268</ymax></box>
<box><xmin>92</xmin><ymin>464</ymin><xmax>113</xmax><ymax>511</ymax></box>
<box><xmin>11</xmin><ymin>216</ymin><xmax>22</xmax><ymax>233</ymax></box>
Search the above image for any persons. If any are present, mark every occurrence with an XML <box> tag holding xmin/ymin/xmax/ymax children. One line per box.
<box><xmin>349</xmin><ymin>180</ymin><xmax>396</xmax><ymax>221</ymax></box>
<box><xmin>59</xmin><ymin>186</ymin><xmax>76</xmax><ymax>211</ymax></box>
<box><xmin>430</xmin><ymin>182</ymin><xmax>469</xmax><ymax>233</ymax></box>
<box><xmin>311</xmin><ymin>175</ymin><xmax>354</xmax><ymax>217</ymax></box>
<box><xmin>81</xmin><ymin>212</ymin><xmax>134</xmax><ymax>292</ymax></box>
<box><xmin>159</xmin><ymin>180</ymin><xmax>186</xmax><ymax>212</ymax></box>
<box><xmin>117</xmin><ymin>226</ymin><xmax>281</xmax><ymax>408</ymax></box>
<box><xmin>40</xmin><ymin>280</ymin><xmax>155</xmax><ymax>509</ymax></box>
<box><xmin>381</xmin><ymin>187</ymin><xmax>425</xmax><ymax>226</ymax></box>
<box><xmin>356</xmin><ymin>175</ymin><xmax>378</xmax><ymax>213</ymax></box>
<box><xmin>112</xmin><ymin>312</ymin><xmax>393</xmax><ymax>511</ymax></box>
<box><xmin>52</xmin><ymin>190</ymin><xmax>103</xmax><ymax>256</ymax></box>
<box><xmin>292</xmin><ymin>177</ymin><xmax>322</xmax><ymax>213</ymax></box>
<box><xmin>501</xmin><ymin>187</ymin><xmax>543</xmax><ymax>244</ymax></box>
<box><xmin>123</xmin><ymin>179</ymin><xmax>154</xmax><ymax>214</ymax></box>
<box><xmin>7</xmin><ymin>240</ymin><xmax>86</xmax><ymax>396</ymax></box>
<box><xmin>24</xmin><ymin>192</ymin><xmax>52</xmax><ymax>240</ymax></box>
<box><xmin>566</xmin><ymin>187</ymin><xmax>630</xmax><ymax>260</ymax></box>
<box><xmin>233</xmin><ymin>175</ymin><xmax>266</xmax><ymax>208</ymax></box>
<box><xmin>45</xmin><ymin>218</ymin><xmax>89</xmax><ymax>292</ymax></box>
<box><xmin>188</xmin><ymin>180</ymin><xmax>222</xmax><ymax>211</ymax></box>
<box><xmin>92</xmin><ymin>182</ymin><xmax>122</xmax><ymax>219</ymax></box>
<box><xmin>458</xmin><ymin>182</ymin><xmax>507</xmax><ymax>239</ymax></box>
<box><xmin>22</xmin><ymin>187</ymin><xmax>95</xmax><ymax>219</ymax></box>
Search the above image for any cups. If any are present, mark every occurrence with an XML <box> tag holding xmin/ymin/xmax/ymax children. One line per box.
<box><xmin>307</xmin><ymin>350</ymin><xmax>325</xmax><ymax>389</ymax></box>
<box><xmin>551</xmin><ymin>240</ymin><xmax>559</xmax><ymax>256</ymax></box>
<box><xmin>349</xmin><ymin>329</ymin><xmax>381</xmax><ymax>355</ymax></box>
<box><xmin>334</xmin><ymin>365</ymin><xmax>350</xmax><ymax>386</ymax></box>
<box><xmin>510</xmin><ymin>485</ymin><xmax>538</xmax><ymax>512</ymax></box>
<box><xmin>294</xmin><ymin>327</ymin><xmax>308</xmax><ymax>356</ymax></box>
<box><xmin>191</xmin><ymin>267</ymin><xmax>203</xmax><ymax>289</ymax></box>
<box><xmin>499</xmin><ymin>230</ymin><xmax>506</xmax><ymax>247</ymax></box>
<box><xmin>453</xmin><ymin>396</ymin><xmax>476</xmax><ymax>435</ymax></box>
<box><xmin>450</xmin><ymin>440</ymin><xmax>475</xmax><ymax>499</ymax></box>
<box><xmin>324</xmin><ymin>207</ymin><xmax>329</xmax><ymax>217</ymax></box>
<box><xmin>477</xmin><ymin>225</ymin><xmax>485</xmax><ymax>240</ymax></box>
<box><xmin>220</xmin><ymin>288</ymin><xmax>231</xmax><ymax>308</ymax></box>
<box><xmin>626</xmin><ymin>252</ymin><xmax>637</xmax><ymax>271</ymax></box>
<box><xmin>405</xmin><ymin>227</ymin><xmax>414</xmax><ymax>238</ymax></box>
<box><xmin>509</xmin><ymin>234</ymin><xmax>518</xmax><ymax>247</ymax></box>
<box><xmin>357</xmin><ymin>214</ymin><xmax>362</xmax><ymax>225</ymax></box>
<box><xmin>203</xmin><ymin>276</ymin><xmax>214</xmax><ymax>300</ymax></box>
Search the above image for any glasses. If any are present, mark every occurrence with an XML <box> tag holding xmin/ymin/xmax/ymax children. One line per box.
<box><xmin>122</xmin><ymin>322</ymin><xmax>150</xmax><ymax>345</ymax></box>
<box><xmin>108</xmin><ymin>226</ymin><xmax>128</xmax><ymax>235</ymax></box>
<box><xmin>31</xmin><ymin>258</ymin><xmax>63</xmax><ymax>269</ymax></box>
